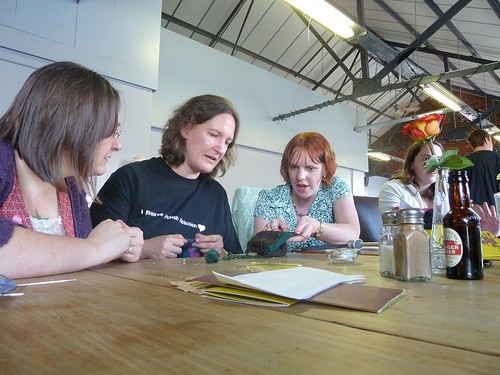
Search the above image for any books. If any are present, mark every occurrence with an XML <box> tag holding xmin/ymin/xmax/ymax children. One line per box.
<box><xmin>192</xmin><ymin>262</ymin><xmax>406</xmax><ymax>313</ymax></box>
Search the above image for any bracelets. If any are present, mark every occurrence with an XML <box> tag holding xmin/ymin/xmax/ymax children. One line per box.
<box><xmin>315</xmin><ymin>221</ymin><xmax>323</xmax><ymax>240</ymax></box>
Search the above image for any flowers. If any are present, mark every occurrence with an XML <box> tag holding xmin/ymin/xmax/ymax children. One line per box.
<box><xmin>402</xmin><ymin>112</ymin><xmax>474</xmax><ymax>194</ymax></box>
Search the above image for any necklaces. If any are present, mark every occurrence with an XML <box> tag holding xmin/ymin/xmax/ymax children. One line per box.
<box><xmin>291</xmin><ymin>194</ymin><xmax>307</xmax><ymax>217</ymax></box>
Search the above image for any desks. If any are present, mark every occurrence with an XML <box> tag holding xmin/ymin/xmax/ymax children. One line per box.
<box><xmin>0</xmin><ymin>245</ymin><xmax>500</xmax><ymax>375</ymax></box>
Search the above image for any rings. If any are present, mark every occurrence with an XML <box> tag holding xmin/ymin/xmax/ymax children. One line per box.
<box><xmin>126</xmin><ymin>247</ymin><xmax>131</xmax><ymax>254</ymax></box>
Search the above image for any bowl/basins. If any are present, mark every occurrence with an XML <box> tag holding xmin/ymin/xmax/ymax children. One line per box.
<box><xmin>324</xmin><ymin>248</ymin><xmax>360</xmax><ymax>263</ymax></box>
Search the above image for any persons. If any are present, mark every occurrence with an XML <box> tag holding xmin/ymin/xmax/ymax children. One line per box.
<box><xmin>0</xmin><ymin>61</ymin><xmax>144</xmax><ymax>279</ymax></box>
<box><xmin>379</xmin><ymin>139</ymin><xmax>500</xmax><ymax>238</ymax></box>
<box><xmin>253</xmin><ymin>132</ymin><xmax>360</xmax><ymax>251</ymax></box>
<box><xmin>89</xmin><ymin>95</ymin><xmax>243</xmax><ymax>260</ymax></box>
<box><xmin>461</xmin><ymin>130</ymin><xmax>500</xmax><ymax>210</ymax></box>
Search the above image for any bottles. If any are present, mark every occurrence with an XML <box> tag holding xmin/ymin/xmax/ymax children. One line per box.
<box><xmin>378</xmin><ymin>211</ymin><xmax>397</xmax><ymax>278</ymax></box>
<box><xmin>393</xmin><ymin>208</ymin><xmax>432</xmax><ymax>282</ymax></box>
<box><xmin>442</xmin><ymin>169</ymin><xmax>484</xmax><ymax>280</ymax></box>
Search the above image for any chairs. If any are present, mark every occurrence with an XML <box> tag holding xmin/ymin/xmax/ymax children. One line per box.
<box><xmin>231</xmin><ymin>187</ymin><xmax>266</xmax><ymax>252</ymax></box>
<box><xmin>353</xmin><ymin>196</ymin><xmax>382</xmax><ymax>244</ymax></box>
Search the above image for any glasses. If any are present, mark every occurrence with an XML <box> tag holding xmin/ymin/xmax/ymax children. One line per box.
<box><xmin>113</xmin><ymin>130</ymin><xmax>121</xmax><ymax>142</ymax></box>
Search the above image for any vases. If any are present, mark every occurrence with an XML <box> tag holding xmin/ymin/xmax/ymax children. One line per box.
<box><xmin>430</xmin><ymin>167</ymin><xmax>449</xmax><ymax>274</ymax></box>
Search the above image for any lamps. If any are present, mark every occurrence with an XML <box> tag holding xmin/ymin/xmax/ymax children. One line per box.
<box><xmin>410</xmin><ymin>1</ymin><xmax>500</xmax><ymax>142</ymax></box>
<box><xmin>285</xmin><ymin>0</ymin><xmax>367</xmax><ymax>41</ymax></box>
